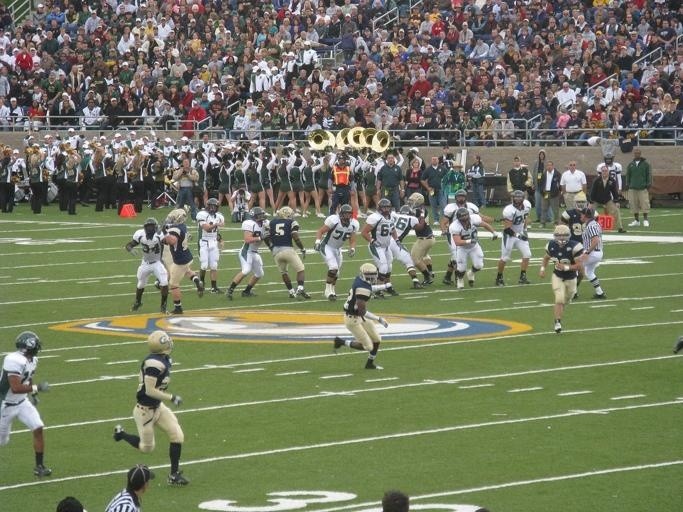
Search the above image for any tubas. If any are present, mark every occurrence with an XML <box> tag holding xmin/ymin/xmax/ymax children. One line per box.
<box><xmin>308</xmin><ymin>126</ymin><xmax>391</xmax><ymax>153</ymax></box>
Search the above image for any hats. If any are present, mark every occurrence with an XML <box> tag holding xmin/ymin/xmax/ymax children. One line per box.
<box><xmin>127</xmin><ymin>464</ymin><xmax>154</xmax><ymax>484</ymax></box>
<box><xmin>57</xmin><ymin>497</ymin><xmax>82</xmax><ymax>512</ymax></box>
<box><xmin>579</xmin><ymin>208</ymin><xmax>590</xmax><ymax>214</ymax></box>
<box><xmin>383</xmin><ymin>493</ymin><xmax>408</xmax><ymax>512</ymax></box>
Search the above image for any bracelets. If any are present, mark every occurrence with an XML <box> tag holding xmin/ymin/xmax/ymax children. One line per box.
<box><xmin>31</xmin><ymin>384</ymin><xmax>38</xmax><ymax>394</ymax></box>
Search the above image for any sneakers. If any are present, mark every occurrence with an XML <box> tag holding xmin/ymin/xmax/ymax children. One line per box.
<box><xmin>34</xmin><ymin>467</ymin><xmax>51</xmax><ymax>476</ymax></box>
<box><xmin>197</xmin><ymin>281</ymin><xmax>203</xmax><ymax>296</ymax></box>
<box><xmin>374</xmin><ymin>287</ymin><xmax>399</xmax><ymax>299</ymax></box>
<box><xmin>642</xmin><ymin>220</ymin><xmax>648</xmax><ymax>226</ymax></box>
<box><xmin>413</xmin><ymin>274</ymin><xmax>434</xmax><ymax>288</ymax></box>
<box><xmin>170</xmin><ymin>308</ymin><xmax>182</xmax><ymax>314</ymax></box>
<box><xmin>167</xmin><ymin>472</ymin><xmax>188</xmax><ymax>486</ymax></box>
<box><xmin>209</xmin><ymin>287</ymin><xmax>223</xmax><ymax>294</ymax></box>
<box><xmin>618</xmin><ymin>228</ymin><xmax>625</xmax><ymax>233</ymax></box>
<box><xmin>225</xmin><ymin>289</ymin><xmax>233</xmax><ymax>300</ymax></box>
<box><xmin>518</xmin><ymin>276</ymin><xmax>529</xmax><ymax>283</ymax></box>
<box><xmin>496</xmin><ymin>278</ymin><xmax>504</xmax><ymax>287</ymax></box>
<box><xmin>288</xmin><ymin>290</ymin><xmax>310</xmax><ymax>298</ymax></box>
<box><xmin>627</xmin><ymin>220</ymin><xmax>639</xmax><ymax>226</ymax></box>
<box><xmin>334</xmin><ymin>337</ymin><xmax>340</xmax><ymax>354</ymax></box>
<box><xmin>324</xmin><ymin>290</ymin><xmax>337</xmax><ymax>301</ymax></box>
<box><xmin>130</xmin><ymin>302</ymin><xmax>142</xmax><ymax>312</ymax></box>
<box><xmin>241</xmin><ymin>291</ymin><xmax>257</xmax><ymax>297</ymax></box>
<box><xmin>554</xmin><ymin>323</ymin><xmax>560</xmax><ymax>333</ymax></box>
<box><xmin>365</xmin><ymin>365</ymin><xmax>382</xmax><ymax>370</ymax></box>
<box><xmin>114</xmin><ymin>425</ymin><xmax>123</xmax><ymax>441</ymax></box>
<box><xmin>442</xmin><ymin>272</ymin><xmax>474</xmax><ymax>288</ymax></box>
<box><xmin>591</xmin><ymin>292</ymin><xmax>606</xmax><ymax>299</ymax></box>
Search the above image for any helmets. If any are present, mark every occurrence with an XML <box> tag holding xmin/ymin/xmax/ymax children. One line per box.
<box><xmin>553</xmin><ymin>225</ymin><xmax>570</xmax><ymax>245</ymax></box>
<box><xmin>573</xmin><ymin>193</ymin><xmax>586</xmax><ymax>201</ymax></box>
<box><xmin>205</xmin><ymin>198</ymin><xmax>218</xmax><ymax>212</ymax></box>
<box><xmin>511</xmin><ymin>190</ymin><xmax>524</xmax><ymax>207</ymax></box>
<box><xmin>148</xmin><ymin>330</ymin><xmax>172</xmax><ymax>355</ymax></box>
<box><xmin>359</xmin><ymin>263</ymin><xmax>378</xmax><ymax>284</ymax></box>
<box><xmin>338</xmin><ymin>204</ymin><xmax>353</xmax><ymax>217</ymax></box>
<box><xmin>454</xmin><ymin>189</ymin><xmax>466</xmax><ymax>204</ymax></box>
<box><xmin>376</xmin><ymin>198</ymin><xmax>391</xmax><ymax>215</ymax></box>
<box><xmin>603</xmin><ymin>152</ymin><xmax>613</xmax><ymax>164</ymax></box>
<box><xmin>15</xmin><ymin>331</ymin><xmax>40</xmax><ymax>355</ymax></box>
<box><xmin>456</xmin><ymin>208</ymin><xmax>469</xmax><ymax>222</ymax></box>
<box><xmin>167</xmin><ymin>209</ymin><xmax>186</xmax><ymax>227</ymax></box>
<box><xmin>279</xmin><ymin>207</ymin><xmax>293</xmax><ymax>219</ymax></box>
<box><xmin>249</xmin><ymin>206</ymin><xmax>266</xmax><ymax>221</ymax></box>
<box><xmin>400</xmin><ymin>192</ymin><xmax>424</xmax><ymax>216</ymax></box>
<box><xmin>143</xmin><ymin>219</ymin><xmax>157</xmax><ymax>234</ymax></box>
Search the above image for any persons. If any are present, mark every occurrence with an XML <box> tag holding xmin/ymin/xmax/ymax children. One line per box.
<box><xmin>56</xmin><ymin>496</ymin><xmax>87</xmax><ymax>512</ymax></box>
<box><xmin>539</xmin><ymin>190</ymin><xmax>607</xmax><ymax>333</ymax></box>
<box><xmin>381</xmin><ymin>489</ymin><xmax>409</xmax><ymax>512</ymax></box>
<box><xmin>113</xmin><ymin>328</ymin><xmax>190</xmax><ymax>486</ymax></box>
<box><xmin>226</xmin><ymin>204</ymin><xmax>359</xmax><ymax>301</ymax></box>
<box><xmin>362</xmin><ymin>189</ymin><xmax>532</xmax><ymax>300</ymax></box>
<box><xmin>126</xmin><ymin>198</ymin><xmax>224</xmax><ymax>317</ymax></box>
<box><xmin>0</xmin><ymin>329</ymin><xmax>53</xmax><ymax>479</ymax></box>
<box><xmin>104</xmin><ymin>463</ymin><xmax>156</xmax><ymax>512</ymax></box>
<box><xmin>475</xmin><ymin>508</ymin><xmax>489</xmax><ymax>512</ymax></box>
<box><xmin>334</xmin><ymin>263</ymin><xmax>388</xmax><ymax>370</ymax></box>
<box><xmin>1</xmin><ymin>1</ymin><xmax>682</xmax><ymax>226</ymax></box>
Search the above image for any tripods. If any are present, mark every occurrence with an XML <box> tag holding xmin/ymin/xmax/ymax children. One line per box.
<box><xmin>148</xmin><ymin>168</ymin><xmax>176</xmax><ymax>207</ymax></box>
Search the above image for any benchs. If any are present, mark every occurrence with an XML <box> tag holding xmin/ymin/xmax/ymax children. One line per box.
<box><xmin>315</xmin><ymin>38</ymin><xmax>342</xmax><ymax>58</ymax></box>
